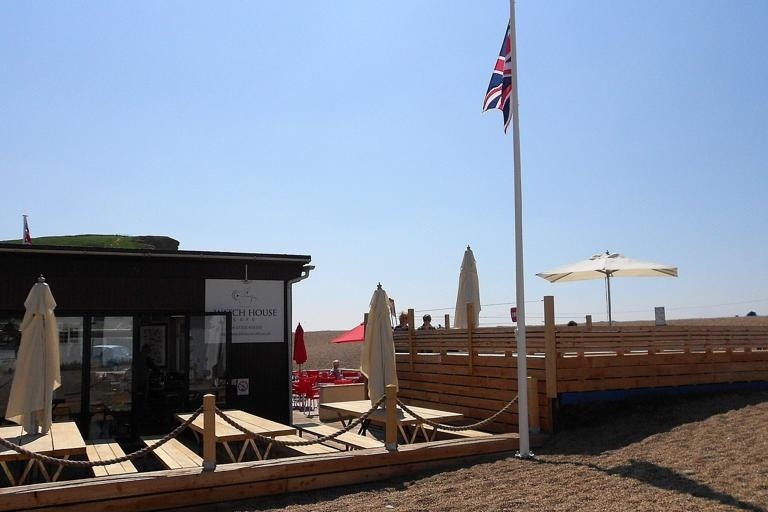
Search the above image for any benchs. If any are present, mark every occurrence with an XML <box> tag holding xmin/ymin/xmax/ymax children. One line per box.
<box><xmin>139</xmin><ymin>434</ymin><xmax>204</xmax><ymax>470</ymax></box>
<box><xmin>84</xmin><ymin>438</ymin><xmax>139</xmax><ymax>477</ymax></box>
<box><xmin>265</xmin><ymin>434</ymin><xmax>340</xmax><ymax>455</ymax></box>
<box><xmin>290</xmin><ymin>423</ymin><xmax>386</xmax><ymax>451</ymax></box>
<box><xmin>411</xmin><ymin>422</ymin><xmax>493</xmax><ymax>438</ymax></box>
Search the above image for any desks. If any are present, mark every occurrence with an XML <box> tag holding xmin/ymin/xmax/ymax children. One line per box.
<box><xmin>319</xmin><ymin>400</ymin><xmax>465</xmax><ymax>445</ymax></box>
<box><xmin>0</xmin><ymin>421</ymin><xmax>86</xmax><ymax>487</ymax></box>
<box><xmin>176</xmin><ymin>410</ymin><xmax>296</xmax><ymax>464</ymax></box>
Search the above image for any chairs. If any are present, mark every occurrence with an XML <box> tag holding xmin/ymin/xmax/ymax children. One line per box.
<box><xmin>292</xmin><ymin>369</ymin><xmax>370</xmax><ymax>418</ymax></box>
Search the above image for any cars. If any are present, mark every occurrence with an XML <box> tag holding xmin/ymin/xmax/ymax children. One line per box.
<box><xmin>90</xmin><ymin>345</ymin><xmax>132</xmax><ymax>373</ymax></box>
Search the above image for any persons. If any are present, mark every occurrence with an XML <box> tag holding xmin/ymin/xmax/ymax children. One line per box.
<box><xmin>138</xmin><ymin>343</ymin><xmax>154</xmax><ymax>408</ymax></box>
<box><xmin>329</xmin><ymin>359</ymin><xmax>343</xmax><ymax>380</ymax></box>
<box><xmin>394</xmin><ymin>314</ymin><xmax>410</xmax><ymax>353</ymax></box>
<box><xmin>416</xmin><ymin>314</ymin><xmax>437</xmax><ymax>353</ymax></box>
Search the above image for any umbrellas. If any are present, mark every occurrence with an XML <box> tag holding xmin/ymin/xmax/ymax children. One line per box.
<box><xmin>329</xmin><ymin>321</ymin><xmax>365</xmax><ymax>343</ymax></box>
<box><xmin>535</xmin><ymin>251</ymin><xmax>679</xmax><ymax>326</ymax></box>
<box><xmin>452</xmin><ymin>245</ymin><xmax>482</xmax><ymax>328</ymax></box>
<box><xmin>360</xmin><ymin>281</ymin><xmax>400</xmax><ymax>408</ymax></box>
<box><xmin>293</xmin><ymin>321</ymin><xmax>308</xmax><ymax>374</ymax></box>
<box><xmin>4</xmin><ymin>275</ymin><xmax>64</xmax><ymax>437</ymax></box>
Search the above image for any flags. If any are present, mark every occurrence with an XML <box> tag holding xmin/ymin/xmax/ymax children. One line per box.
<box><xmin>481</xmin><ymin>18</ymin><xmax>514</xmax><ymax>134</ymax></box>
